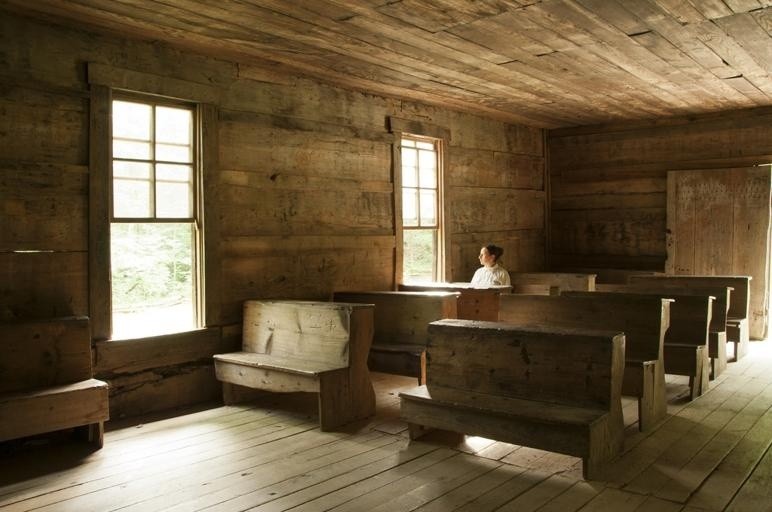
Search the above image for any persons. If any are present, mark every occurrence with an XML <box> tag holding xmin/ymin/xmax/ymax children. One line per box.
<box><xmin>470</xmin><ymin>245</ymin><xmax>511</xmax><ymax>287</ymax></box>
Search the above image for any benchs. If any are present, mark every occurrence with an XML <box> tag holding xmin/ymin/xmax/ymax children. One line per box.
<box><xmin>214</xmin><ymin>296</ymin><xmax>378</xmax><ymax>431</ymax></box>
<box><xmin>396</xmin><ymin>317</ymin><xmax>631</xmax><ymax>479</ymax></box>
<box><xmin>640</xmin><ymin>284</ymin><xmax>736</xmax><ymax>381</ymax></box>
<box><xmin>633</xmin><ymin>293</ymin><xmax>718</xmax><ymax>401</ymax></box>
<box><xmin>0</xmin><ymin>315</ymin><xmax>110</xmax><ymax>449</ymax></box>
<box><xmin>461</xmin><ymin>266</ymin><xmax>597</xmax><ymax>297</ymax></box>
<box><xmin>334</xmin><ymin>289</ymin><xmax>461</xmax><ymax>386</ymax></box>
<box><xmin>494</xmin><ymin>291</ymin><xmax>677</xmax><ymax>435</ymax></box>
<box><xmin>627</xmin><ymin>271</ymin><xmax>751</xmax><ymax>364</ymax></box>
<box><xmin>400</xmin><ymin>278</ymin><xmax>512</xmax><ymax>321</ymax></box>
